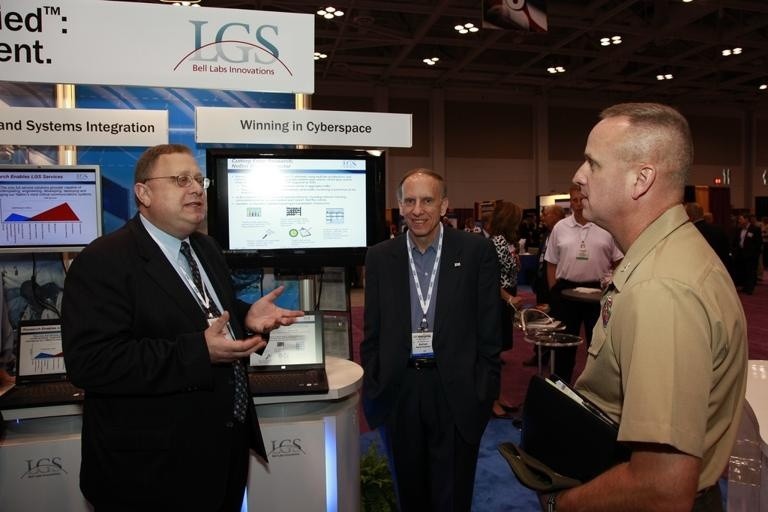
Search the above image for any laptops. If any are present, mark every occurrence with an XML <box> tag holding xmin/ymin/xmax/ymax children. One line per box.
<box><xmin>246</xmin><ymin>311</ymin><xmax>329</xmax><ymax>397</ymax></box>
<box><xmin>0</xmin><ymin>319</ymin><xmax>84</xmax><ymax>410</ymax></box>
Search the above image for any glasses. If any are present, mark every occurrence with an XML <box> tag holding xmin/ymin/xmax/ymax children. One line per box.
<box><xmin>144</xmin><ymin>173</ymin><xmax>211</xmax><ymax>189</ymax></box>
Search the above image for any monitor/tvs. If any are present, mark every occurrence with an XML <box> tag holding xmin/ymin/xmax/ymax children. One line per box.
<box><xmin>0</xmin><ymin>165</ymin><xmax>103</xmax><ymax>254</ymax></box>
<box><xmin>205</xmin><ymin>147</ymin><xmax>386</xmax><ymax>282</ymax></box>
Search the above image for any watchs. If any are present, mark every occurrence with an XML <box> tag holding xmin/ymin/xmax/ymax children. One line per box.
<box><xmin>547</xmin><ymin>492</ymin><xmax>559</xmax><ymax>512</ymax></box>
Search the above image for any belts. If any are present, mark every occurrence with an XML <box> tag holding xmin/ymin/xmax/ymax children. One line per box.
<box><xmin>414</xmin><ymin>358</ymin><xmax>434</xmax><ymax>367</ymax></box>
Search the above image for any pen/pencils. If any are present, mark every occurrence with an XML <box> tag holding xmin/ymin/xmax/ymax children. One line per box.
<box><xmin>582</xmin><ymin>401</ymin><xmax>619</xmax><ymax>429</ymax></box>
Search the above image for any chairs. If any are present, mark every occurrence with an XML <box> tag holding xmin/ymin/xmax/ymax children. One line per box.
<box><xmin>512</xmin><ymin>308</ymin><xmax>584</xmax><ymax>433</ymax></box>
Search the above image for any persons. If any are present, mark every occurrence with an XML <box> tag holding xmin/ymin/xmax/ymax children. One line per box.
<box><xmin>760</xmin><ymin>216</ymin><xmax>768</xmax><ymax>270</ymax></box>
<box><xmin>679</xmin><ymin>199</ymin><xmax>733</xmax><ymax>274</ymax></box>
<box><xmin>542</xmin><ymin>103</ymin><xmax>747</xmax><ymax>511</ymax></box>
<box><xmin>361</xmin><ymin>167</ymin><xmax>507</xmax><ymax>512</ymax></box>
<box><xmin>484</xmin><ymin>202</ymin><xmax>528</xmax><ymax>418</ymax></box>
<box><xmin>748</xmin><ymin>215</ymin><xmax>764</xmax><ymax>282</ymax></box>
<box><xmin>519</xmin><ymin>208</ymin><xmax>532</xmax><ymax>252</ymax></box>
<box><xmin>59</xmin><ymin>144</ymin><xmax>304</xmax><ymax>512</ymax></box>
<box><xmin>733</xmin><ymin>212</ymin><xmax>761</xmax><ymax>295</ymax></box>
<box><xmin>542</xmin><ymin>183</ymin><xmax>624</xmax><ymax>384</ymax></box>
<box><xmin>522</xmin><ymin>205</ymin><xmax>565</xmax><ymax>367</ymax></box>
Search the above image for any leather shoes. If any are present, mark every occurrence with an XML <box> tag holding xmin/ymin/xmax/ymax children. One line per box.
<box><xmin>500</xmin><ymin>400</ymin><xmax>522</xmax><ymax>413</ymax></box>
<box><xmin>522</xmin><ymin>356</ymin><xmax>547</xmax><ymax>367</ymax></box>
<box><xmin>490</xmin><ymin>404</ymin><xmax>513</xmax><ymax>419</ymax></box>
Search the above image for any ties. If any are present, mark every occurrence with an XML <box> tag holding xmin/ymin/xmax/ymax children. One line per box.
<box><xmin>180</xmin><ymin>240</ymin><xmax>248</xmax><ymax>424</ymax></box>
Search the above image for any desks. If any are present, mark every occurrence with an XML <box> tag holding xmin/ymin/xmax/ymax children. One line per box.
<box><xmin>0</xmin><ymin>355</ymin><xmax>365</xmax><ymax>512</ymax></box>
<box><xmin>745</xmin><ymin>360</ymin><xmax>768</xmax><ymax>512</ymax></box>
<box><xmin>561</xmin><ymin>288</ymin><xmax>603</xmax><ymax>301</ymax></box>
